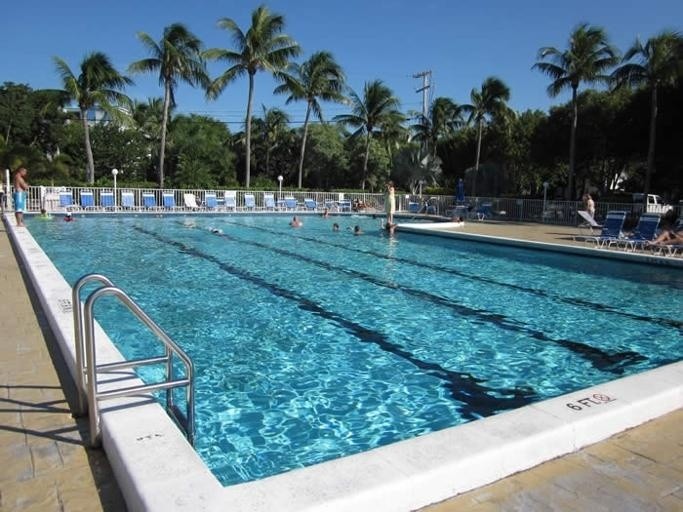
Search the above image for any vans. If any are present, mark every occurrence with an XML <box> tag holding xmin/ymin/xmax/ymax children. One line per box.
<box><xmin>609</xmin><ymin>190</ymin><xmax>674</xmax><ymax>220</ymax></box>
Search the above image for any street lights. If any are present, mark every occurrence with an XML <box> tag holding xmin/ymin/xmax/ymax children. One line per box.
<box><xmin>277</xmin><ymin>175</ymin><xmax>284</xmax><ymax>200</ymax></box>
<box><xmin>542</xmin><ymin>181</ymin><xmax>549</xmax><ymax>212</ymax></box>
<box><xmin>112</xmin><ymin>168</ymin><xmax>118</xmax><ymax>213</ymax></box>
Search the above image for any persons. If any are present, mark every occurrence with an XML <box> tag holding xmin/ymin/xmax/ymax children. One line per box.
<box><xmin>198</xmin><ymin>206</ymin><xmax>398</xmax><ymax>239</ymax></box>
<box><xmin>13</xmin><ymin>166</ymin><xmax>28</xmax><ymax>227</ymax></box>
<box><xmin>644</xmin><ymin>207</ymin><xmax>683</xmax><ymax>246</ymax></box>
<box><xmin>62</xmin><ymin>212</ymin><xmax>72</xmax><ymax>222</ymax></box>
<box><xmin>582</xmin><ymin>194</ymin><xmax>595</xmax><ymax>219</ymax></box>
<box><xmin>34</xmin><ymin>209</ymin><xmax>56</xmax><ymax>222</ymax></box>
<box><xmin>382</xmin><ymin>180</ymin><xmax>396</xmax><ymax>225</ymax></box>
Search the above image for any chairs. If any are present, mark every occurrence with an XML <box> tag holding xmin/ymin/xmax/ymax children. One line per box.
<box><xmin>58</xmin><ymin>190</ymin><xmax>352</xmax><ymax>213</ymax></box>
<box><xmin>409</xmin><ymin>196</ymin><xmax>437</xmax><ymax>216</ymax></box>
<box><xmin>570</xmin><ymin>210</ymin><xmax>683</xmax><ymax>257</ymax></box>
<box><xmin>445</xmin><ymin>198</ymin><xmax>512</xmax><ymax>221</ymax></box>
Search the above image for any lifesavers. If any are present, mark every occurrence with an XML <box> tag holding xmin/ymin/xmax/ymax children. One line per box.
<box><xmin>34</xmin><ymin>215</ymin><xmax>56</xmax><ymax>221</ymax></box>
<box><xmin>64</xmin><ymin>218</ymin><xmax>75</xmax><ymax>222</ymax></box>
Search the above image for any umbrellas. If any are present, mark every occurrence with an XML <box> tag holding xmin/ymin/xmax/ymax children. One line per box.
<box><xmin>455</xmin><ymin>179</ymin><xmax>465</xmax><ymax>205</ymax></box>
